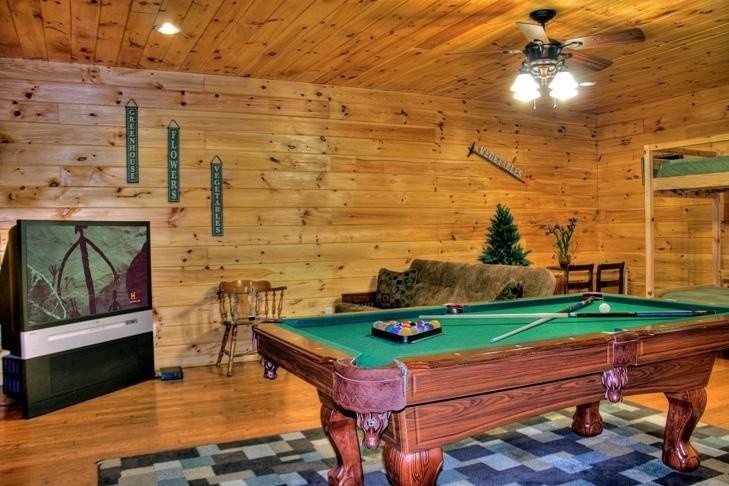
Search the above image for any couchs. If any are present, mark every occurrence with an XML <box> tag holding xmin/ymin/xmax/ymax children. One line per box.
<box><xmin>335</xmin><ymin>256</ymin><xmax>557</xmax><ymax>317</ymax></box>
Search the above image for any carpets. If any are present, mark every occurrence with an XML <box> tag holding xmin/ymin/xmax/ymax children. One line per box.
<box><xmin>96</xmin><ymin>395</ymin><xmax>728</xmax><ymax>486</ymax></box>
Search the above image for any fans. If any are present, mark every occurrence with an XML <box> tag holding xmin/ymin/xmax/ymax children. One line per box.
<box><xmin>444</xmin><ymin>8</ymin><xmax>646</xmax><ymax>71</ymax></box>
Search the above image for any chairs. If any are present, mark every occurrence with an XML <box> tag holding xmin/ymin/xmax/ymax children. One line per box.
<box><xmin>215</xmin><ymin>279</ymin><xmax>287</xmax><ymax>378</ymax></box>
<box><xmin>560</xmin><ymin>263</ymin><xmax>594</xmax><ymax>294</ymax></box>
<box><xmin>596</xmin><ymin>261</ymin><xmax>625</xmax><ymax>295</ymax></box>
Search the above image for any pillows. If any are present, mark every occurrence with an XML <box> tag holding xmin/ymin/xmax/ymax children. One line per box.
<box><xmin>495</xmin><ymin>279</ymin><xmax>523</xmax><ymax>302</ymax></box>
<box><xmin>374</xmin><ymin>267</ymin><xmax>417</xmax><ymax>310</ymax></box>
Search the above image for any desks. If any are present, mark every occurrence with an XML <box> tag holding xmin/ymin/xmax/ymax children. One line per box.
<box><xmin>252</xmin><ymin>291</ymin><xmax>728</xmax><ymax>485</ymax></box>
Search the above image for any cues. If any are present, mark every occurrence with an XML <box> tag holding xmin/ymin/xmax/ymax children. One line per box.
<box><xmin>490</xmin><ymin>297</ymin><xmax>594</xmax><ymax>343</ymax></box>
<box><xmin>419</xmin><ymin>310</ymin><xmax>716</xmax><ymax>318</ymax></box>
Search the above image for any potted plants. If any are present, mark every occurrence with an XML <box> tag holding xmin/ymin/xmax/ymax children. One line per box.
<box><xmin>543</xmin><ymin>218</ymin><xmax>580</xmax><ymax>267</ymax></box>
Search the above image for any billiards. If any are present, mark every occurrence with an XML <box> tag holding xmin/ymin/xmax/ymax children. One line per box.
<box><xmin>602</xmin><ymin>372</ymin><xmax>629</xmax><ymax>402</ymax></box>
<box><xmin>599</xmin><ymin>303</ymin><xmax>610</xmax><ymax>313</ymax></box>
<box><xmin>373</xmin><ymin>320</ymin><xmax>440</xmax><ymax>336</ymax></box>
<box><xmin>363</xmin><ymin>415</ymin><xmax>381</xmax><ymax>449</ymax></box>
<box><xmin>265</xmin><ymin>361</ymin><xmax>276</xmax><ymax>379</ymax></box>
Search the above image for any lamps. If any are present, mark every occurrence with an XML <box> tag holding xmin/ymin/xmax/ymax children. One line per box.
<box><xmin>507</xmin><ymin>71</ymin><xmax>580</xmax><ymax>108</ymax></box>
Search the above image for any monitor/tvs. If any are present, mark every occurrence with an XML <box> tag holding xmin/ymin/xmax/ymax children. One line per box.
<box><xmin>0</xmin><ymin>218</ymin><xmax>155</xmax><ymax>360</ymax></box>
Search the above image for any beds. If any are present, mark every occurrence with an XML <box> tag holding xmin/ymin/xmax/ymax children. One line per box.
<box><xmin>639</xmin><ymin>133</ymin><xmax>729</xmax><ymax>361</ymax></box>
